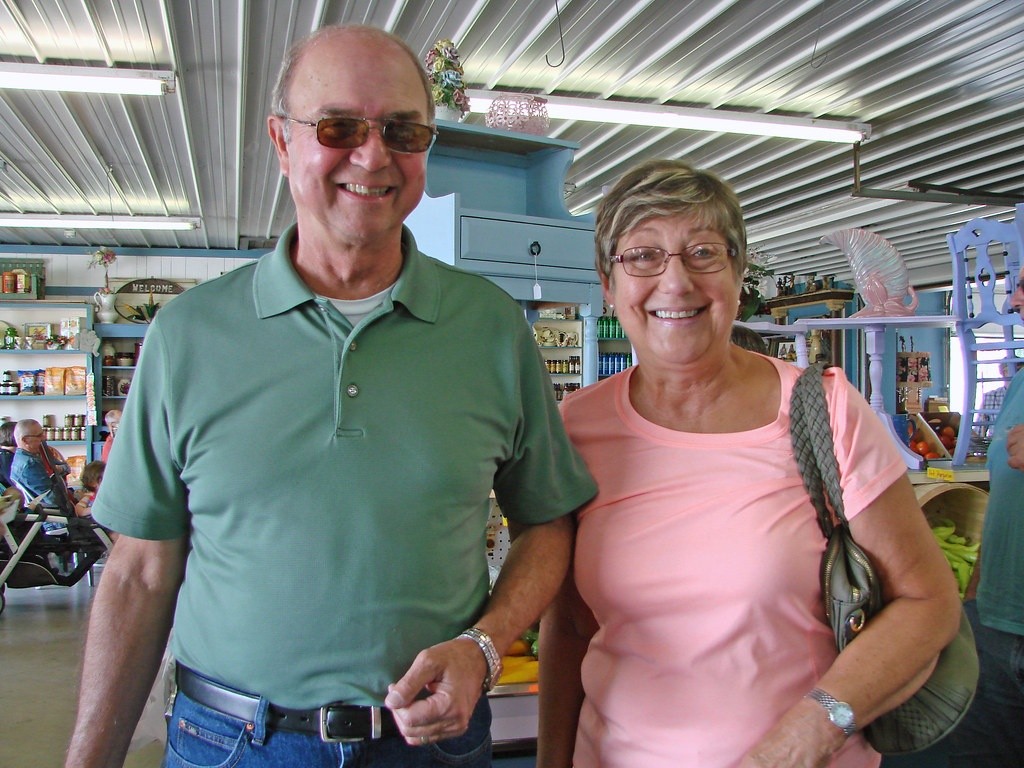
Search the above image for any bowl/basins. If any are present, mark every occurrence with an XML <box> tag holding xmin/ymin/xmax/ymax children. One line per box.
<box><xmin>115</xmin><ymin>351</ymin><xmax>135</xmax><ymax>366</ymax></box>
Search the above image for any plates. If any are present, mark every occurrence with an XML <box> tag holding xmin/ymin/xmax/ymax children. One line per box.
<box><xmin>0</xmin><ymin>320</ymin><xmax>10</xmax><ymax>349</ymax></box>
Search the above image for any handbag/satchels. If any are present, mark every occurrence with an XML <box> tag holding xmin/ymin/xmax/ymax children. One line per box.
<box><xmin>787</xmin><ymin>359</ymin><xmax>981</xmax><ymax>756</ymax></box>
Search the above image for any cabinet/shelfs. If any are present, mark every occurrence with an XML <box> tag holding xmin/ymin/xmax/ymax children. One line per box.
<box><xmin>535</xmin><ymin>317</ymin><xmax>583</xmax><ymax>404</ymax></box>
<box><xmin>93</xmin><ymin>320</ymin><xmax>153</xmax><ymax>463</ymax></box>
<box><xmin>0</xmin><ymin>302</ymin><xmax>95</xmax><ymax>490</ymax></box>
<box><xmin>600</xmin><ymin>337</ymin><xmax>629</xmax><ymax>380</ymax></box>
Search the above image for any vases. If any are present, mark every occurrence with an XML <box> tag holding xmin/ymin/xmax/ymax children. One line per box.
<box><xmin>93</xmin><ymin>293</ymin><xmax>118</xmax><ymax>324</ymax></box>
<box><xmin>433</xmin><ymin>105</ymin><xmax>463</xmax><ymax>121</ymax></box>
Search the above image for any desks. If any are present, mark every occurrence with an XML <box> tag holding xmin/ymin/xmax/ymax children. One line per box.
<box><xmin>792</xmin><ymin>315</ymin><xmax>964</xmax><ymax>470</ymax></box>
<box><xmin>732</xmin><ymin>320</ymin><xmax>809</xmax><ymax>370</ymax></box>
<box><xmin>403</xmin><ymin>119</ymin><xmax>604</xmax><ymax>386</ymax></box>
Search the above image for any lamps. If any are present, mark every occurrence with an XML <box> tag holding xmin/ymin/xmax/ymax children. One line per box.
<box><xmin>0</xmin><ymin>213</ymin><xmax>202</xmax><ymax>231</ymax></box>
<box><xmin>0</xmin><ymin>61</ymin><xmax>178</xmax><ymax>98</ymax></box>
<box><xmin>464</xmin><ymin>82</ymin><xmax>873</xmax><ymax>145</ymax></box>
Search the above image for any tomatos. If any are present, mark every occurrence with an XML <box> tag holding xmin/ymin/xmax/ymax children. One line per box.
<box><xmin>909</xmin><ymin>425</ymin><xmax>957</xmax><ymax>460</ymax></box>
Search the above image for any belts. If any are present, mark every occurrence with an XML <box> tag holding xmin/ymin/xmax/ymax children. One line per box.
<box><xmin>175</xmin><ymin>659</ymin><xmax>414</xmax><ymax>743</ymax></box>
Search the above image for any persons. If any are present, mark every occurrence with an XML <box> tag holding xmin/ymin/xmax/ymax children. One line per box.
<box><xmin>534</xmin><ymin>160</ymin><xmax>962</xmax><ymax>768</ymax></box>
<box><xmin>974</xmin><ymin>355</ymin><xmax>1024</xmax><ymax>455</ymax></box>
<box><xmin>946</xmin><ymin>259</ymin><xmax>1024</xmax><ymax>768</ymax></box>
<box><xmin>67</xmin><ymin>23</ymin><xmax>601</xmax><ymax>768</ymax></box>
<box><xmin>0</xmin><ymin>409</ymin><xmax>123</xmax><ymax>543</ymax></box>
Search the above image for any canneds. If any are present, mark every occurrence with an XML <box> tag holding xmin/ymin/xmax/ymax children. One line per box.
<box><xmin>102</xmin><ymin>375</ymin><xmax>115</xmax><ymax>396</ymax></box>
<box><xmin>0</xmin><ymin>370</ymin><xmax>19</xmax><ymax>395</ymax></box>
<box><xmin>4</xmin><ymin>275</ymin><xmax>14</xmax><ymax>294</ymax></box>
<box><xmin>41</xmin><ymin>413</ymin><xmax>86</xmax><ymax>440</ymax></box>
<box><xmin>544</xmin><ymin>359</ymin><xmax>569</xmax><ymax>374</ymax></box>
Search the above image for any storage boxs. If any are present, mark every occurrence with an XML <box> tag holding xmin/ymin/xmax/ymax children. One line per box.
<box><xmin>0</xmin><ymin>259</ymin><xmax>46</xmax><ymax>300</ymax></box>
<box><xmin>915</xmin><ymin>411</ymin><xmax>979</xmax><ymax>459</ymax></box>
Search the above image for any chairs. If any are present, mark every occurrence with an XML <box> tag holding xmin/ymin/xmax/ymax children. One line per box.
<box><xmin>945</xmin><ymin>202</ymin><xmax>1024</xmax><ymax>470</ymax></box>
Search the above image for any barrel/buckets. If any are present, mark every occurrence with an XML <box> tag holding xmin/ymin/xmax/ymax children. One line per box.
<box><xmin>909</xmin><ymin>483</ymin><xmax>990</xmax><ymax>604</ymax></box>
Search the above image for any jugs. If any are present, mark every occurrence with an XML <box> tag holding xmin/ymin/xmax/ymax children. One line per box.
<box><xmin>566</xmin><ymin>332</ymin><xmax>578</xmax><ymax>348</ymax></box>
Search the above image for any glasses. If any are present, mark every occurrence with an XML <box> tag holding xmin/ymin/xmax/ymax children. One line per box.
<box><xmin>286</xmin><ymin>117</ymin><xmax>440</xmax><ymax>154</ymax></box>
<box><xmin>609</xmin><ymin>243</ymin><xmax>737</xmax><ymax>277</ymax></box>
<box><xmin>24</xmin><ymin>431</ymin><xmax>44</xmax><ymax>437</ymax></box>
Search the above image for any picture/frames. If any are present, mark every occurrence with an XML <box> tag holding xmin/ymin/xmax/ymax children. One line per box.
<box><xmin>25</xmin><ymin>322</ymin><xmax>51</xmax><ymax>338</ymax></box>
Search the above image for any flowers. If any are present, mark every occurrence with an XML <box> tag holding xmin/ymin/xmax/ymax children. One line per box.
<box><xmin>85</xmin><ymin>247</ymin><xmax>120</xmax><ymax>295</ymax></box>
<box><xmin>425</xmin><ymin>36</ymin><xmax>472</xmax><ymax>111</ymax></box>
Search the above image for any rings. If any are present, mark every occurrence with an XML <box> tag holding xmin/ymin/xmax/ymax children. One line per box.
<box><xmin>421</xmin><ymin>736</ymin><xmax>429</xmax><ymax>745</ymax></box>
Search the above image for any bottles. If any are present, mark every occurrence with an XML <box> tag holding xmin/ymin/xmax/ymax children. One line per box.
<box><xmin>101</xmin><ymin>342</ymin><xmax>117</xmax><ymax>366</ymax></box>
<box><xmin>596</xmin><ymin>315</ymin><xmax>627</xmax><ymax>339</ymax></box>
<box><xmin>543</xmin><ymin>355</ymin><xmax>581</xmax><ymax>374</ymax></box>
<box><xmin>3</xmin><ymin>327</ymin><xmax>18</xmax><ymax>350</ymax></box>
<box><xmin>552</xmin><ymin>382</ymin><xmax>580</xmax><ymax>401</ymax></box>
<box><xmin>117</xmin><ymin>377</ymin><xmax>131</xmax><ymax>396</ymax></box>
<box><xmin>598</xmin><ymin>351</ymin><xmax>632</xmax><ymax>375</ymax></box>
<box><xmin>102</xmin><ymin>375</ymin><xmax>115</xmax><ymax>397</ymax></box>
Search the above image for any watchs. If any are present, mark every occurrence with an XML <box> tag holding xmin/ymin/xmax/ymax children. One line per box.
<box><xmin>457</xmin><ymin>626</ymin><xmax>504</xmax><ymax>692</ymax></box>
<box><xmin>804</xmin><ymin>688</ymin><xmax>856</xmax><ymax>736</ymax></box>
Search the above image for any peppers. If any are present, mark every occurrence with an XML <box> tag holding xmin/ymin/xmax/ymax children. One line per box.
<box><xmin>927</xmin><ymin>517</ymin><xmax>981</xmax><ymax>597</ymax></box>
